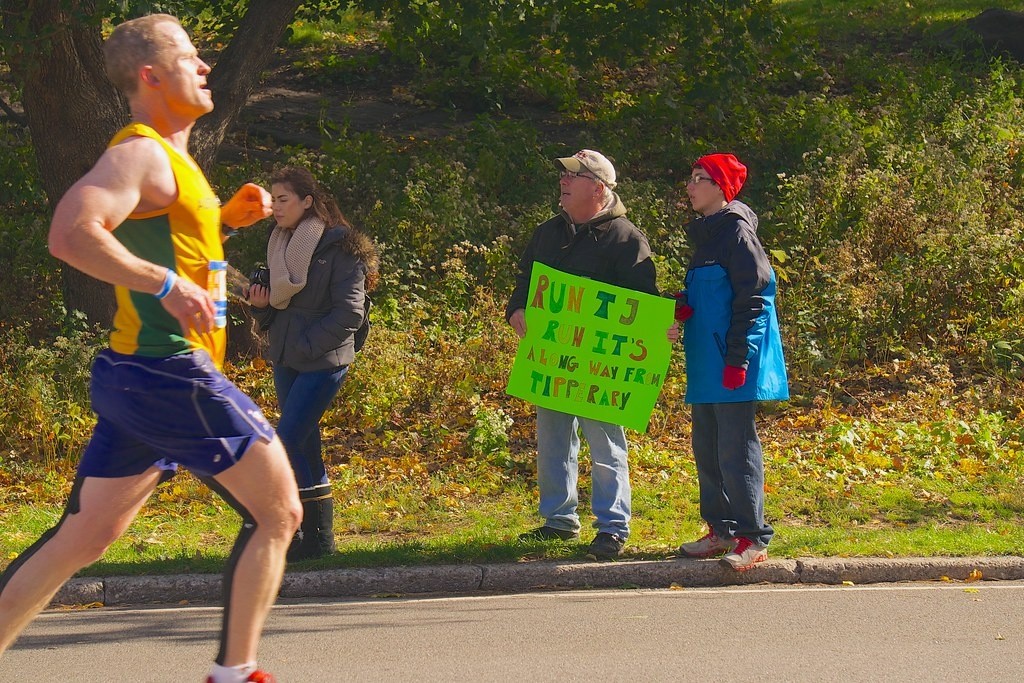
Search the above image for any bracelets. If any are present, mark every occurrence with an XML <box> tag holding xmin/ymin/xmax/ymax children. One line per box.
<box><xmin>154</xmin><ymin>268</ymin><xmax>177</xmax><ymax>300</ymax></box>
<box><xmin>221</xmin><ymin>222</ymin><xmax>239</xmax><ymax>237</ymax></box>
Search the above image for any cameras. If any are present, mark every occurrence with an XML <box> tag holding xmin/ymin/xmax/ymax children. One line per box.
<box><xmin>249</xmin><ymin>262</ymin><xmax>271</xmax><ymax>290</ymax></box>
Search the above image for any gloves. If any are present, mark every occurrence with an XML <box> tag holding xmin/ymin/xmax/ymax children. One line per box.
<box><xmin>722</xmin><ymin>365</ymin><xmax>746</xmax><ymax>391</ymax></box>
<box><xmin>674</xmin><ymin>292</ymin><xmax>693</xmax><ymax>322</ymax></box>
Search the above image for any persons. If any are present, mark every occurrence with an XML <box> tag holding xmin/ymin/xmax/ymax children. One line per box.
<box><xmin>506</xmin><ymin>150</ymin><xmax>680</xmax><ymax>556</ymax></box>
<box><xmin>673</xmin><ymin>154</ymin><xmax>789</xmax><ymax>570</ymax></box>
<box><xmin>248</xmin><ymin>166</ymin><xmax>370</xmax><ymax>561</ymax></box>
<box><xmin>0</xmin><ymin>14</ymin><xmax>304</xmax><ymax>683</ymax></box>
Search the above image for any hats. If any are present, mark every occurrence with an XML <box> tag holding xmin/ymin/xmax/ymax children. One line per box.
<box><xmin>693</xmin><ymin>154</ymin><xmax>747</xmax><ymax>203</ymax></box>
<box><xmin>553</xmin><ymin>149</ymin><xmax>616</xmax><ymax>190</ymax></box>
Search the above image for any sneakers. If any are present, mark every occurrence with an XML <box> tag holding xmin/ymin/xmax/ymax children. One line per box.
<box><xmin>680</xmin><ymin>526</ymin><xmax>739</xmax><ymax>559</ymax></box>
<box><xmin>519</xmin><ymin>525</ymin><xmax>580</xmax><ymax>543</ymax></box>
<box><xmin>717</xmin><ymin>538</ymin><xmax>767</xmax><ymax>572</ymax></box>
<box><xmin>587</xmin><ymin>533</ymin><xmax>625</xmax><ymax>561</ymax></box>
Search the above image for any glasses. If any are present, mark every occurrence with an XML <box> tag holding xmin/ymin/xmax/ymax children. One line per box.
<box><xmin>689</xmin><ymin>175</ymin><xmax>713</xmax><ymax>184</ymax></box>
<box><xmin>559</xmin><ymin>171</ymin><xmax>594</xmax><ymax>182</ymax></box>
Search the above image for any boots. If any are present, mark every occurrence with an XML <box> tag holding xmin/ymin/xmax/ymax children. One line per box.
<box><xmin>286</xmin><ymin>482</ymin><xmax>335</xmax><ymax>562</ymax></box>
